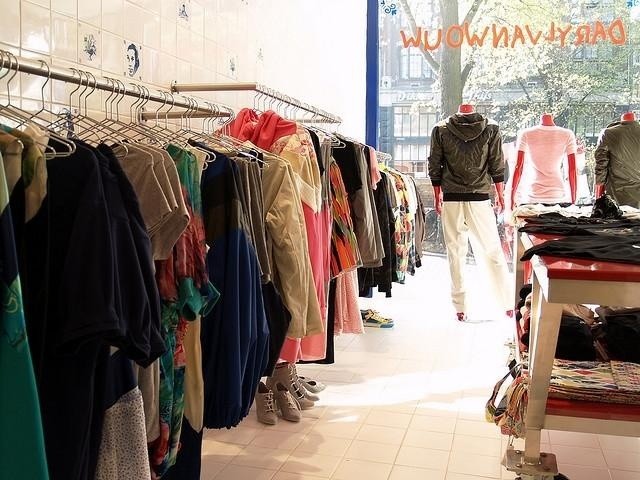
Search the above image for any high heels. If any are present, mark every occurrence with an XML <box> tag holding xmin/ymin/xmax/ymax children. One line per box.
<box><xmin>256</xmin><ymin>361</ymin><xmax>325</xmax><ymax>425</ymax></box>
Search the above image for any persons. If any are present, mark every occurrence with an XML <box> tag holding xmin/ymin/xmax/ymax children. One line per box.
<box><xmin>507</xmin><ymin>114</ymin><xmax>581</xmax><ymax>317</ymax></box>
<box><xmin>590</xmin><ymin>110</ymin><xmax>639</xmax><ymax>210</ymax></box>
<box><xmin>124</xmin><ymin>43</ymin><xmax>142</xmax><ymax>77</ymax></box>
<box><xmin>428</xmin><ymin>98</ymin><xmax>514</xmax><ymax>323</ymax></box>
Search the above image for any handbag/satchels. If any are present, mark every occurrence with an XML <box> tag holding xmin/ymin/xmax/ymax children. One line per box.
<box><xmin>485</xmin><ymin>357</ymin><xmax>640</xmax><ymax>438</ymax></box>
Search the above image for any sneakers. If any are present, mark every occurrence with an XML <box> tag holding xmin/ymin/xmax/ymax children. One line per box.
<box><xmin>360</xmin><ymin>308</ymin><xmax>394</xmax><ymax>326</ymax></box>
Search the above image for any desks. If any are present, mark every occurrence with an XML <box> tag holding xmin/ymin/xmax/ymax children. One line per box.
<box><xmin>508</xmin><ymin>208</ymin><xmax>640</xmax><ymax>480</ymax></box>
